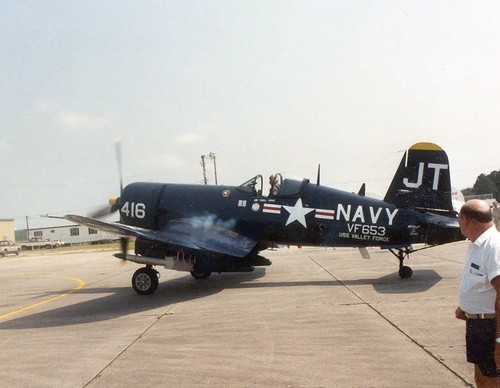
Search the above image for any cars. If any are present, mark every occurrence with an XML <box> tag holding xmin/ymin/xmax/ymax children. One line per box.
<box><xmin>0</xmin><ymin>240</ymin><xmax>21</xmax><ymax>257</ymax></box>
<box><xmin>19</xmin><ymin>237</ymin><xmax>57</xmax><ymax>251</ymax></box>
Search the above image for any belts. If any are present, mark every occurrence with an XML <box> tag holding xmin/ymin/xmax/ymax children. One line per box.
<box><xmin>462</xmin><ymin>312</ymin><xmax>497</xmax><ymax>319</ymax></box>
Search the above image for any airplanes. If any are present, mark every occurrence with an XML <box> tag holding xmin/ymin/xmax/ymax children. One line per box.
<box><xmin>62</xmin><ymin>141</ymin><xmax>468</xmax><ymax>296</ymax></box>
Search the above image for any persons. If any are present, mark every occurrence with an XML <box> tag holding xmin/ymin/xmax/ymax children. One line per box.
<box><xmin>454</xmin><ymin>199</ymin><xmax>500</xmax><ymax>388</ymax></box>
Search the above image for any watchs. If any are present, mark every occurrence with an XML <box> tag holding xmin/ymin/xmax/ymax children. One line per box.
<box><xmin>493</xmin><ymin>337</ymin><xmax>500</xmax><ymax>343</ymax></box>
